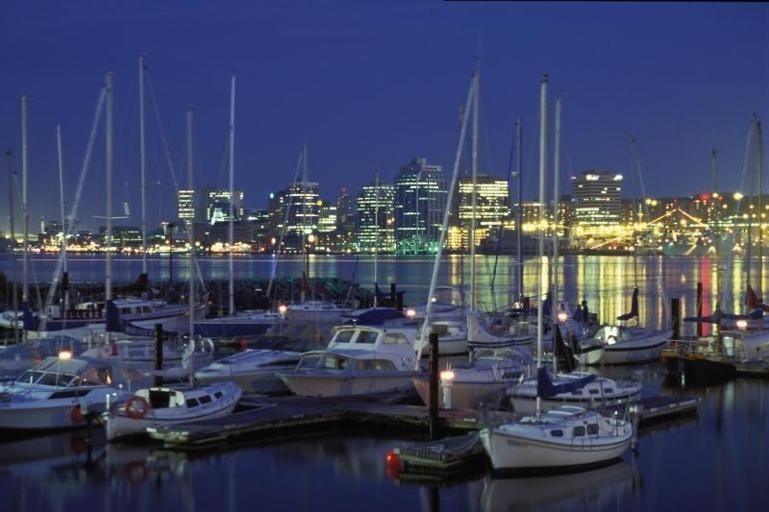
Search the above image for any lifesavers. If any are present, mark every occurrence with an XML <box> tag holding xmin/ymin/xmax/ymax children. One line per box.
<box><xmin>202</xmin><ymin>337</ymin><xmax>214</xmax><ymax>355</ymax></box>
<box><xmin>126</xmin><ymin>395</ymin><xmax>148</xmax><ymax>419</ymax></box>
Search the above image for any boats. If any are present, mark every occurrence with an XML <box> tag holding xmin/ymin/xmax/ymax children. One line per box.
<box><xmin>660</xmin><ymin>333</ymin><xmax>737</xmax><ymax>390</ymax></box>
<box><xmin>479</xmin><ymin>461</ymin><xmax>635</xmax><ymax>512</ymax></box>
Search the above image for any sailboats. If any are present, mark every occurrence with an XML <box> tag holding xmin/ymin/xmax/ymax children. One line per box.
<box><xmin>473</xmin><ymin>68</ymin><xmax>635</xmax><ymax>477</ymax></box>
<box><xmin>681</xmin><ymin>109</ymin><xmax>768</xmax><ymax>369</ymax></box>
<box><xmin>0</xmin><ymin>55</ymin><xmax>675</xmax><ymax>448</ymax></box>
